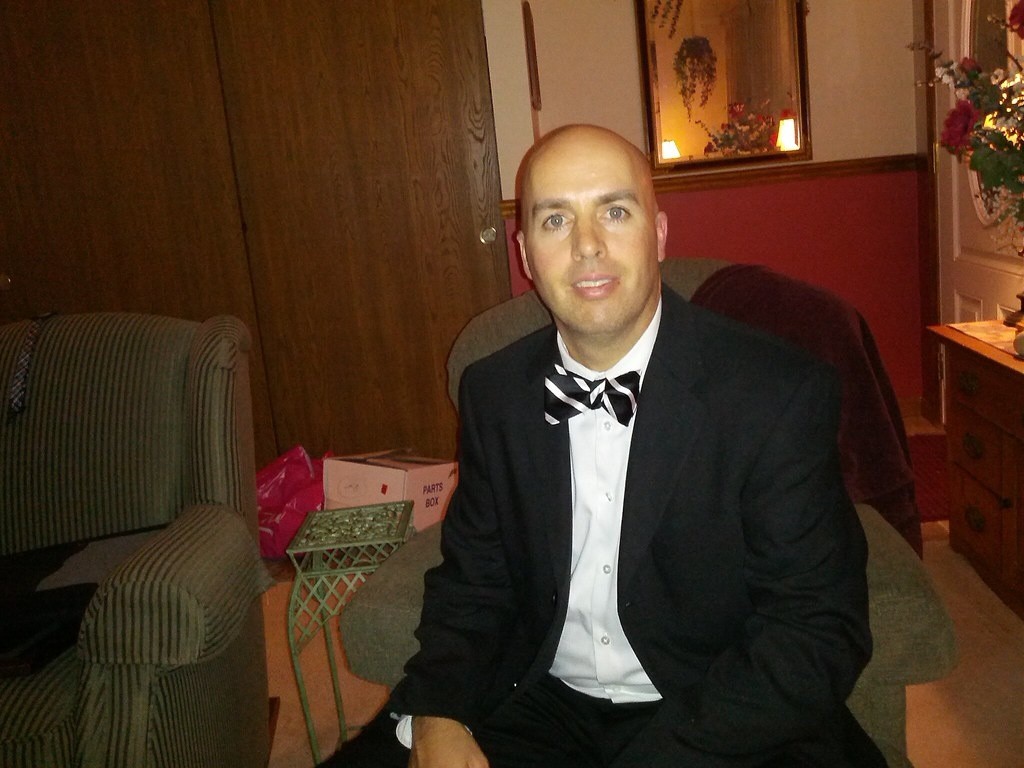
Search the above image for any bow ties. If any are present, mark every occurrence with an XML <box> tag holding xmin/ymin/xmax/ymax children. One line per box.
<box><xmin>544</xmin><ymin>363</ymin><xmax>642</xmax><ymax>426</ymax></box>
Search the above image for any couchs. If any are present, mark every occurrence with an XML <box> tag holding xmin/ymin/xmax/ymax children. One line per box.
<box><xmin>0</xmin><ymin>313</ymin><xmax>271</xmax><ymax>768</ymax></box>
<box><xmin>339</xmin><ymin>258</ymin><xmax>955</xmax><ymax>768</ymax></box>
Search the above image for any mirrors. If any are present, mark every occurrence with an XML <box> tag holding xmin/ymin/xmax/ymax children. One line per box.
<box><xmin>634</xmin><ymin>0</ymin><xmax>813</xmax><ymax>178</ymax></box>
<box><xmin>960</xmin><ymin>0</ymin><xmax>1013</xmax><ymax>227</ymax></box>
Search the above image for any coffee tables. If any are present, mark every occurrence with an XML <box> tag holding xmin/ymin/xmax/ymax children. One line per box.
<box><xmin>285</xmin><ymin>500</ymin><xmax>417</xmax><ymax>765</ymax></box>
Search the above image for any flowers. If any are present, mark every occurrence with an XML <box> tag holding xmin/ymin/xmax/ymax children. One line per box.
<box><xmin>904</xmin><ymin>0</ymin><xmax>1024</xmax><ymax>256</ymax></box>
<box><xmin>695</xmin><ymin>102</ymin><xmax>796</xmax><ymax>158</ymax></box>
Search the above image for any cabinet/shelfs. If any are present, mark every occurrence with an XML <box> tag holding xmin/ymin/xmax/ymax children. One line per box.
<box><xmin>927</xmin><ymin>318</ymin><xmax>1024</xmax><ymax>621</ymax></box>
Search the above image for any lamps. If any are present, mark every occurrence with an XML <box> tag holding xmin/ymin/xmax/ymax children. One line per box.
<box><xmin>663</xmin><ymin>140</ymin><xmax>681</xmax><ymax>160</ymax></box>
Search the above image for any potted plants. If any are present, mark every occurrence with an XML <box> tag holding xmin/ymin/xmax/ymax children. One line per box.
<box><xmin>673</xmin><ymin>36</ymin><xmax>717</xmax><ymax>123</ymax></box>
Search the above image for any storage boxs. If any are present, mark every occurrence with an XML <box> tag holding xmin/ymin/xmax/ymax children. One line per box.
<box><xmin>324</xmin><ymin>449</ymin><xmax>461</xmax><ymax>531</ymax></box>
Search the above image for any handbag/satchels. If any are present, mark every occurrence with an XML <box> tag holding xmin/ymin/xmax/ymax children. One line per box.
<box><xmin>255</xmin><ymin>444</ymin><xmax>334</xmax><ymax>558</ymax></box>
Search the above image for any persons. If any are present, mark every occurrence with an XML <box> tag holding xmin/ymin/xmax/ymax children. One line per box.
<box><xmin>317</xmin><ymin>124</ymin><xmax>890</xmax><ymax>768</ymax></box>
